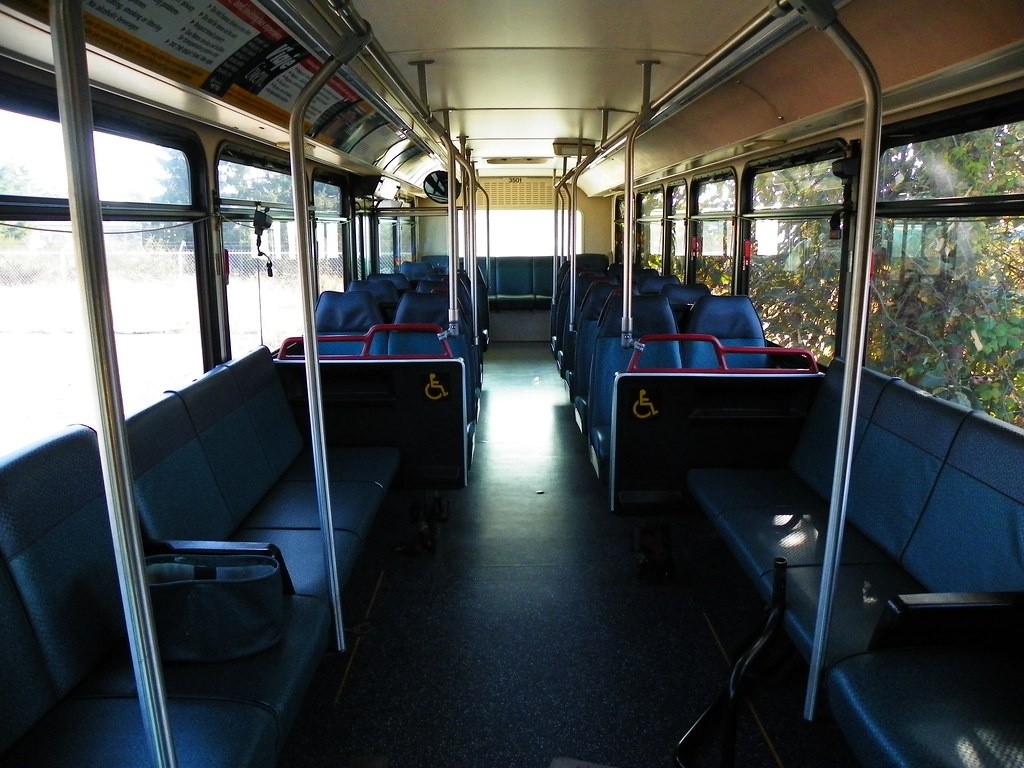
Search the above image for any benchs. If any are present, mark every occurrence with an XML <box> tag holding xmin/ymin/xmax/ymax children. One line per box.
<box><xmin>684</xmin><ymin>355</ymin><xmax>1023</xmax><ymax>768</ymax></box>
<box><xmin>422</xmin><ymin>254</ymin><xmax>609</xmax><ymax>310</ymax></box>
<box><xmin>0</xmin><ymin>345</ymin><xmax>403</xmax><ymax>768</ymax></box>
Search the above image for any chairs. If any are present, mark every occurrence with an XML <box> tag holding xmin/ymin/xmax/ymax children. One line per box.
<box><xmin>547</xmin><ymin>256</ymin><xmax>773</xmax><ymax>481</ymax></box>
<box><xmin>315</xmin><ymin>261</ymin><xmax>492</xmax><ymax>470</ymax></box>
